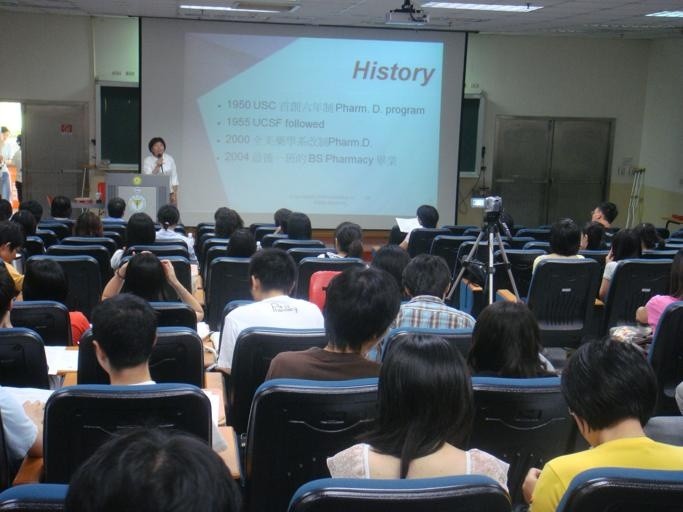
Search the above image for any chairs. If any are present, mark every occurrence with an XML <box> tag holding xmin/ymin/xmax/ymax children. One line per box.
<box><xmin>0</xmin><ymin>219</ymin><xmax>683</xmax><ymax>512</ymax></box>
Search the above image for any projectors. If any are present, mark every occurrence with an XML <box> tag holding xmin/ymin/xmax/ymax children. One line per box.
<box><xmin>385</xmin><ymin>12</ymin><xmax>429</xmax><ymax>25</ymax></box>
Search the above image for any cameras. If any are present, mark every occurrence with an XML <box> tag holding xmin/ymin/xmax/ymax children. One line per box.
<box><xmin>470</xmin><ymin>195</ymin><xmax>502</xmax><ymax>213</ymax></box>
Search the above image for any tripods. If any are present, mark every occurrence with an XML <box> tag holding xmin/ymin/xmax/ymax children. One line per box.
<box><xmin>445</xmin><ymin>213</ymin><xmax>519</xmax><ymax>305</ymax></box>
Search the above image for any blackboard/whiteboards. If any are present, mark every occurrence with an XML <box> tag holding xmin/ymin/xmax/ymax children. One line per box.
<box><xmin>96</xmin><ymin>81</ymin><xmax>486</xmax><ymax>178</ymax></box>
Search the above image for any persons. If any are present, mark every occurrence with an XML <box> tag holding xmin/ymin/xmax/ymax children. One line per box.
<box><xmin>0</xmin><ymin>203</ymin><xmax>683</xmax><ymax>512</ymax></box>
<box><xmin>142</xmin><ymin>137</ymin><xmax>178</xmax><ymax>206</ymax></box>
<box><xmin>1</xmin><ymin>126</ymin><xmax>23</xmax><ymax>211</ymax></box>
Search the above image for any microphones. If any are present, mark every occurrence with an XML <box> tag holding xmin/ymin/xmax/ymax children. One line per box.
<box><xmin>158</xmin><ymin>153</ymin><xmax>163</xmax><ymax>170</ymax></box>
